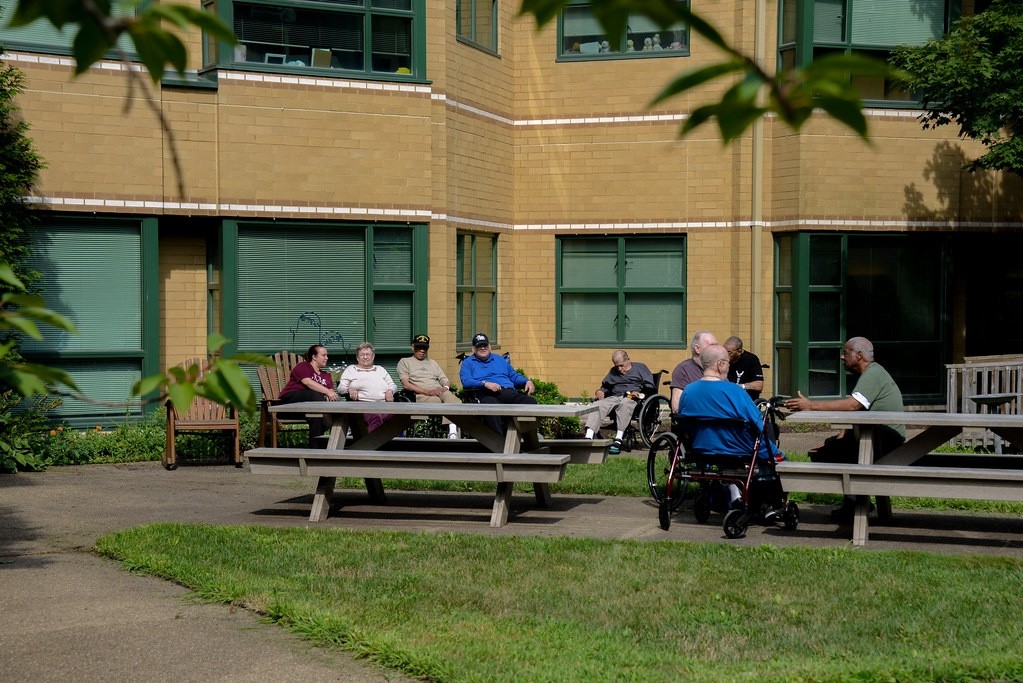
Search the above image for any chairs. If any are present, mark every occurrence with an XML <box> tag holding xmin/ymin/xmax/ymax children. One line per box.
<box><xmin>256</xmin><ymin>351</ymin><xmax>307</xmax><ymax>447</ymax></box>
<box><xmin>163</xmin><ymin>357</ymin><xmax>243</xmax><ymax>470</ymax></box>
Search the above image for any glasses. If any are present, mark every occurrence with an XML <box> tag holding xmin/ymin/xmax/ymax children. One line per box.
<box><xmin>718</xmin><ymin>359</ymin><xmax>732</xmax><ymax>367</ymax></box>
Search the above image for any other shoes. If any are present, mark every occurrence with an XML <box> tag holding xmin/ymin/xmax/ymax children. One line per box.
<box><xmin>731</xmin><ymin>497</ymin><xmax>745</xmax><ymax>510</ymax></box>
<box><xmin>760</xmin><ymin>502</ymin><xmax>785</xmax><ymax>518</ymax></box>
<box><xmin>830</xmin><ymin>495</ymin><xmax>876</xmax><ymax>518</ymax></box>
<box><xmin>447</xmin><ymin>433</ymin><xmax>457</xmax><ymax>439</ymax></box>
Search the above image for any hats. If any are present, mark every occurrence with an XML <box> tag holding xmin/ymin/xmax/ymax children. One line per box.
<box><xmin>412</xmin><ymin>334</ymin><xmax>430</xmax><ymax>347</ymax></box>
<box><xmin>472</xmin><ymin>334</ymin><xmax>488</xmax><ymax>346</ymax></box>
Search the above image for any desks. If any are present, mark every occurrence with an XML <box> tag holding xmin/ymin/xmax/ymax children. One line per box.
<box><xmin>785</xmin><ymin>410</ymin><xmax>1023</xmax><ymax>550</ymax></box>
<box><xmin>269</xmin><ymin>401</ymin><xmax>600</xmax><ymax>527</ymax></box>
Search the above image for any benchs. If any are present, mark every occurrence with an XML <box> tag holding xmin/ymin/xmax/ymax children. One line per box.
<box><xmin>245</xmin><ymin>448</ymin><xmax>570</xmax><ymax>483</ymax></box>
<box><xmin>314</xmin><ymin>434</ymin><xmax>612</xmax><ymax>463</ymax></box>
<box><xmin>807</xmin><ymin>447</ymin><xmax>1023</xmax><ymax>469</ymax></box>
<box><xmin>776</xmin><ymin>461</ymin><xmax>1023</xmax><ymax>502</ymax></box>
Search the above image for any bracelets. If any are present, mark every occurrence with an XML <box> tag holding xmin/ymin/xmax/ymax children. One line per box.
<box><xmin>445</xmin><ymin>386</ymin><xmax>449</xmax><ymax>390</ymax></box>
<box><xmin>742</xmin><ymin>384</ymin><xmax>745</xmax><ymax>389</ymax></box>
<box><xmin>482</xmin><ymin>380</ymin><xmax>486</xmax><ymax>386</ymax></box>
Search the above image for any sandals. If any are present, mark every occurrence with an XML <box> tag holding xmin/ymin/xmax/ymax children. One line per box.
<box><xmin>608</xmin><ymin>439</ymin><xmax>623</xmax><ymax>453</ymax></box>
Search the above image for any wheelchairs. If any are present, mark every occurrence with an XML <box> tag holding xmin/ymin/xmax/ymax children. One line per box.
<box><xmin>601</xmin><ymin>369</ymin><xmax>669</xmax><ymax>452</ymax></box>
<box><xmin>658</xmin><ymin>397</ymin><xmax>800</xmax><ymax>537</ymax></box>
<box><xmin>645</xmin><ymin>432</ymin><xmax>692</xmax><ymax>510</ymax></box>
<box><xmin>758</xmin><ymin>364</ymin><xmax>776</xmax><ymax>430</ymax></box>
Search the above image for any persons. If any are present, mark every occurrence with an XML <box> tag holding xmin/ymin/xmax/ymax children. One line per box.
<box><xmin>724</xmin><ymin>336</ymin><xmax>764</xmax><ymax>402</ymax></box>
<box><xmin>397</xmin><ymin>335</ymin><xmax>462</xmax><ymax>439</ymax></box>
<box><xmin>459</xmin><ymin>333</ymin><xmax>542</xmax><ymax>439</ymax></box>
<box><xmin>679</xmin><ymin>345</ymin><xmax>782</xmax><ymax>510</ymax></box>
<box><xmin>586</xmin><ymin>351</ymin><xmax>654</xmax><ymax>453</ymax></box>
<box><xmin>280</xmin><ymin>345</ymin><xmax>352</xmax><ymax>435</ymax></box>
<box><xmin>784</xmin><ymin>337</ymin><xmax>906</xmax><ymax>518</ymax></box>
<box><xmin>338</xmin><ymin>342</ymin><xmax>398</xmax><ymax>433</ymax></box>
<box><xmin>671</xmin><ymin>332</ymin><xmax>717</xmax><ymax>413</ymax></box>
